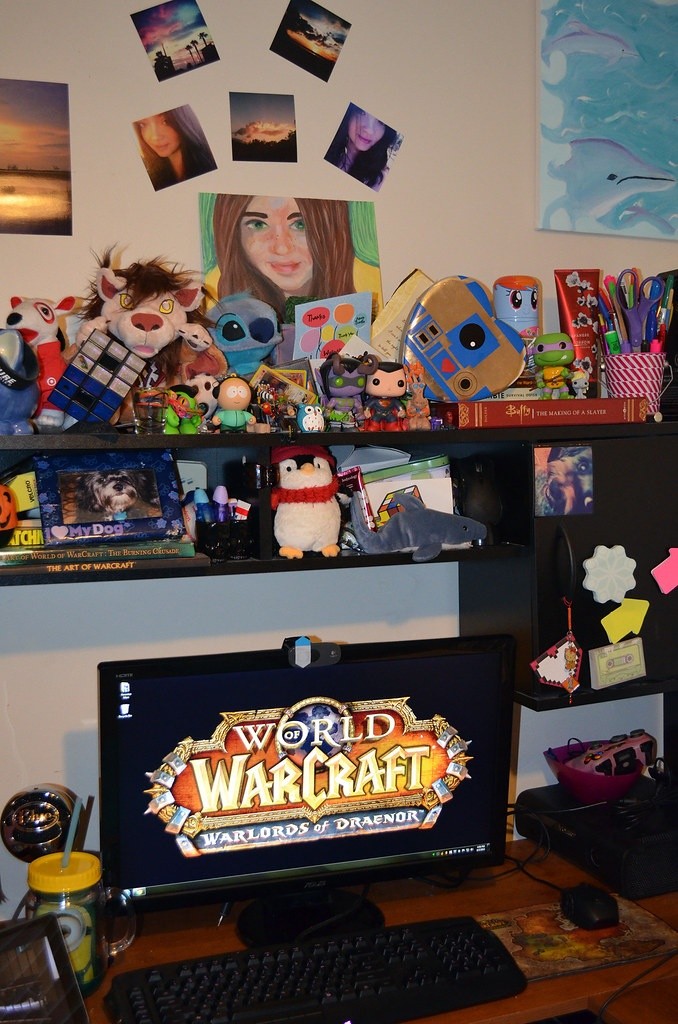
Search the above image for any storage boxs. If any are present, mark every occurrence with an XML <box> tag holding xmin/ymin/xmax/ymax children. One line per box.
<box><xmin>430</xmin><ymin>396</ymin><xmax>648</xmax><ymax>429</ymax></box>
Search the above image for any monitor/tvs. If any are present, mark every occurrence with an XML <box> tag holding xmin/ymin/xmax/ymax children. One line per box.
<box><xmin>98</xmin><ymin>632</ymin><xmax>518</xmax><ymax>949</ymax></box>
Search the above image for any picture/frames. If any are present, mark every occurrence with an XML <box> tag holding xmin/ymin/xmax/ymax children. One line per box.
<box><xmin>33</xmin><ymin>448</ymin><xmax>184</xmax><ymax>546</ymax></box>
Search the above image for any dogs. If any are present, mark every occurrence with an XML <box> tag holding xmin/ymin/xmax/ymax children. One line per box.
<box><xmin>75</xmin><ymin>467</ymin><xmax>148</xmax><ymax>514</ymax></box>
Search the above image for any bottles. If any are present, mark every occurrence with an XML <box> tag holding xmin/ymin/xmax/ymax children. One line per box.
<box><xmin>25</xmin><ymin>851</ymin><xmax>137</xmax><ymax>998</ymax></box>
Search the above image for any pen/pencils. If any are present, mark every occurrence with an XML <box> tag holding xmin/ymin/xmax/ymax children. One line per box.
<box><xmin>599</xmin><ymin>267</ymin><xmax>675</xmax><ymax>355</ymax></box>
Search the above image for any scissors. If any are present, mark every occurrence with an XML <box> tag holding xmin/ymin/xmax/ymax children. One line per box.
<box><xmin>615</xmin><ymin>269</ymin><xmax>664</xmax><ymax>353</ymax></box>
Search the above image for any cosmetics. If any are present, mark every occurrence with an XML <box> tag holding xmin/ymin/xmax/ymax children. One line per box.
<box><xmin>553</xmin><ymin>268</ymin><xmax>600</xmax><ymax>398</ymax></box>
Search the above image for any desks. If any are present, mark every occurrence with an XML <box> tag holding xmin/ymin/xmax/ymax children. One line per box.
<box><xmin>0</xmin><ymin>836</ymin><xmax>678</xmax><ymax>1024</ymax></box>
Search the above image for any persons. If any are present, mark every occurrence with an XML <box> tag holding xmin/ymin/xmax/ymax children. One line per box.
<box><xmin>316</xmin><ymin>353</ymin><xmax>378</xmax><ymax>432</ymax></box>
<box><xmin>324</xmin><ymin>102</ymin><xmax>397</xmax><ymax>193</ymax></box>
<box><xmin>211</xmin><ymin>377</ymin><xmax>256</xmax><ymax>433</ymax></box>
<box><xmin>363</xmin><ymin>361</ymin><xmax>405</xmax><ymax>430</ymax></box>
<box><xmin>132</xmin><ymin>105</ymin><xmax>218</xmax><ymax>192</ymax></box>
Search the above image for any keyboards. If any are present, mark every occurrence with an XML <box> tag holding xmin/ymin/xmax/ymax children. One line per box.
<box><xmin>111</xmin><ymin>915</ymin><xmax>527</xmax><ymax>1024</ymax></box>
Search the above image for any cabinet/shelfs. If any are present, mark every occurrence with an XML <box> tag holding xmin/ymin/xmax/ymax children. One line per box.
<box><xmin>0</xmin><ymin>424</ymin><xmax>678</xmax><ymax>781</ymax></box>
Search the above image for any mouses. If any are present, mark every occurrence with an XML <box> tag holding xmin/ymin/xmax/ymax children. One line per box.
<box><xmin>560</xmin><ymin>882</ymin><xmax>619</xmax><ymax>930</ymax></box>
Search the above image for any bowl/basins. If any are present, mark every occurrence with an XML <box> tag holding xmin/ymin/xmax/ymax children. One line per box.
<box><xmin>543</xmin><ymin>742</ymin><xmax>644</xmax><ymax>805</ymax></box>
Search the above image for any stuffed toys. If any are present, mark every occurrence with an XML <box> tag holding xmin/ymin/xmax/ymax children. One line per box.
<box><xmin>5</xmin><ymin>245</ymin><xmax>283</xmax><ymax>433</ymax></box>
<box><xmin>260</xmin><ymin>443</ymin><xmax>352</xmax><ymax>558</ymax></box>
<box><xmin>351</xmin><ymin>493</ymin><xmax>485</xmax><ymax>562</ymax></box>
<box><xmin>0</xmin><ymin>485</ymin><xmax>17</xmax><ymax>549</ymax></box>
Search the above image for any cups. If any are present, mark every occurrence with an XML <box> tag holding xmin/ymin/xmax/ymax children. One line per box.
<box><xmin>603</xmin><ymin>352</ymin><xmax>666</xmax><ymax>415</ymax></box>
